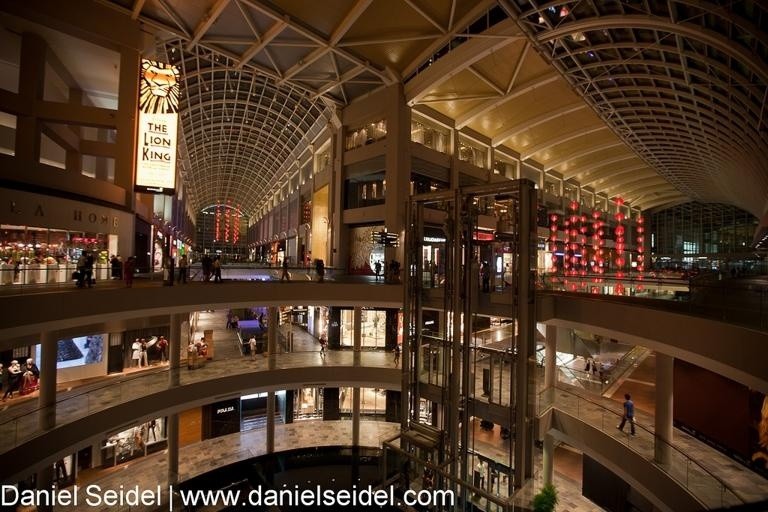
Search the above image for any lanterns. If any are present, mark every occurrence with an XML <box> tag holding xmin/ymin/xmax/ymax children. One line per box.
<box><xmin>215</xmin><ymin>196</ymin><xmax>242</xmax><ymax>245</ymax></box>
<box><xmin>551</xmin><ymin>197</ymin><xmax>645</xmax><ymax>294</ymax></box>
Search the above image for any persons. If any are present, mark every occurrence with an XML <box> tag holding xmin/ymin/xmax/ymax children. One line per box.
<box><xmin>187</xmin><ymin>338</ymin><xmax>208</xmax><ymax>362</ymax></box>
<box><xmin>259</xmin><ymin>312</ymin><xmax>264</xmax><ymax>328</ymax></box>
<box><xmin>319</xmin><ymin>334</ymin><xmax>327</xmax><ymax>355</ymax></box>
<box><xmin>616</xmin><ymin>394</ymin><xmax>636</xmax><ymax>435</ymax></box>
<box><xmin>76</xmin><ymin>250</ymin><xmax>87</xmax><ymax>285</ymax></box>
<box><xmin>145</xmin><ymin>420</ymin><xmax>156</xmax><ymax>441</ymax></box>
<box><xmin>225</xmin><ymin>310</ymin><xmax>239</xmax><ymax>333</ymax></box>
<box><xmin>281</xmin><ymin>257</ymin><xmax>291</xmax><ymax>281</ymax></box>
<box><xmin>480</xmin><ymin>257</ymin><xmax>489</xmax><ymax>293</ymax></box>
<box><xmin>249</xmin><ymin>335</ymin><xmax>257</xmax><ymax>361</ymax></box>
<box><xmin>425</xmin><ymin>258</ymin><xmax>446</xmax><ymax>287</ymax></box>
<box><xmin>157</xmin><ymin>336</ymin><xmax>169</xmax><ymax>362</ymax></box>
<box><xmin>476</xmin><ymin>459</ymin><xmax>486</xmax><ymax>491</ymax></box>
<box><xmin>131</xmin><ymin>338</ymin><xmax>141</xmax><ymax>367</ymax></box>
<box><xmin>392</xmin><ymin>345</ymin><xmax>400</xmax><ymax>368</ymax></box>
<box><xmin>84</xmin><ymin>254</ymin><xmax>96</xmax><ymax>289</ymax></box>
<box><xmin>138</xmin><ymin>339</ymin><xmax>149</xmax><ymax>366</ymax></box>
<box><xmin>22</xmin><ymin>357</ymin><xmax>40</xmax><ymax>388</ymax></box>
<box><xmin>374</xmin><ymin>260</ymin><xmax>400</xmax><ymax>282</ymax></box>
<box><xmin>1</xmin><ymin>359</ymin><xmax>22</xmax><ymax>402</ymax></box>
<box><xmin>584</xmin><ymin>359</ymin><xmax>608</xmax><ymax>383</ymax></box>
<box><xmin>111</xmin><ymin>253</ymin><xmax>223</xmax><ymax>289</ymax></box>
